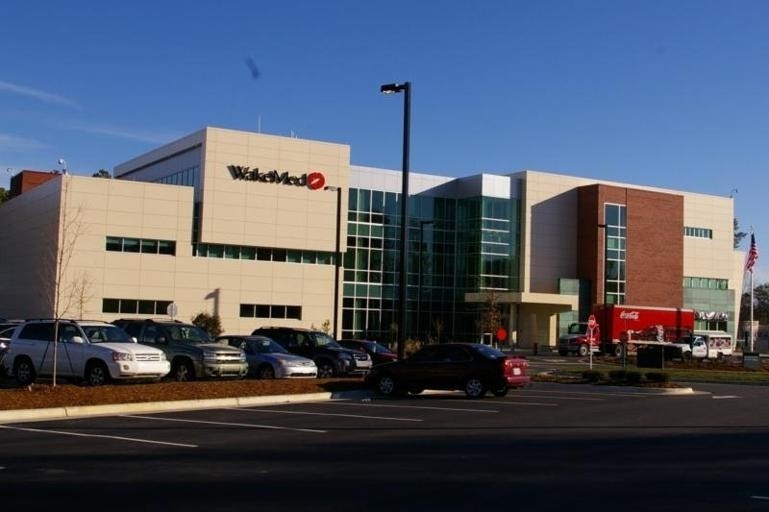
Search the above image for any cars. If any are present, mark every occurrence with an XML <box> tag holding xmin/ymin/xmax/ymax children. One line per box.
<box><xmin>364</xmin><ymin>342</ymin><xmax>531</xmax><ymax>400</ymax></box>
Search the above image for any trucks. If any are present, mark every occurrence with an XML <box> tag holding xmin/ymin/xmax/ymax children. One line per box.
<box><xmin>556</xmin><ymin>303</ymin><xmax>734</xmax><ymax>361</ymax></box>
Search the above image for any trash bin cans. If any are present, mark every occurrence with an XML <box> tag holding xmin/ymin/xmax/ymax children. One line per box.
<box><xmin>743</xmin><ymin>352</ymin><xmax>760</xmax><ymax>370</ymax></box>
<box><xmin>637</xmin><ymin>347</ymin><xmax>657</xmax><ymax>369</ymax></box>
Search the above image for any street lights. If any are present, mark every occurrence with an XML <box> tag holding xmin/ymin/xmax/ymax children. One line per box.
<box><xmin>379</xmin><ymin>81</ymin><xmax>411</xmax><ymax>363</ymax></box>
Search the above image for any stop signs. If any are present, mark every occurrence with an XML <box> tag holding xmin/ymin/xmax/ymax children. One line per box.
<box><xmin>495</xmin><ymin>327</ymin><xmax>508</xmax><ymax>341</ymax></box>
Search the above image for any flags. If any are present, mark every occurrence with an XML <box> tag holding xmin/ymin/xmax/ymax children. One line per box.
<box><xmin>745</xmin><ymin>232</ymin><xmax>758</xmax><ymax>273</ymax></box>
